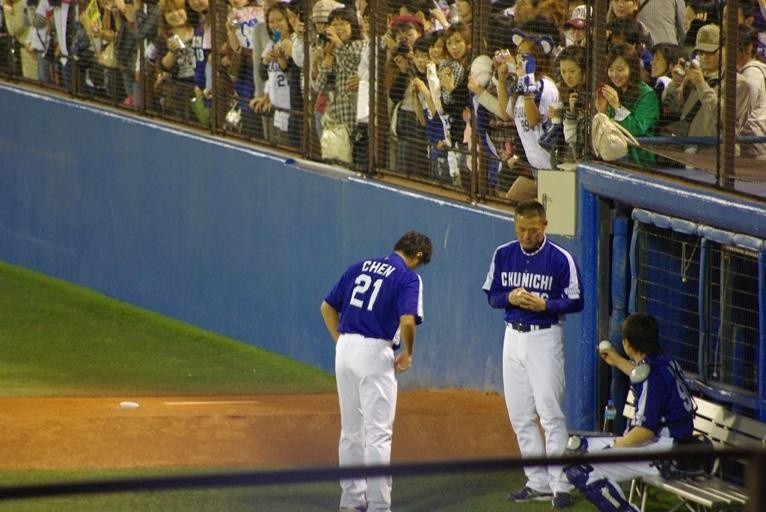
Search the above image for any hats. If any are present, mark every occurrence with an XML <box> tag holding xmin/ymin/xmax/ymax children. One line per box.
<box><xmin>691</xmin><ymin>23</ymin><xmax>721</xmax><ymax>53</ymax></box>
<box><xmin>564</xmin><ymin>4</ymin><xmax>593</xmax><ymax>32</ymax></box>
<box><xmin>311</xmin><ymin>0</ymin><xmax>346</xmax><ymax>23</ymax></box>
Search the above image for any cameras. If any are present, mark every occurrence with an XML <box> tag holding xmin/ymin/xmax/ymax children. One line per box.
<box><xmin>598</xmin><ymin>82</ymin><xmax>605</xmax><ymax>99</ymax></box>
<box><xmin>572</xmin><ymin>91</ymin><xmax>592</xmax><ymax>112</ymax></box>
<box><xmin>679</xmin><ymin>59</ymin><xmax>696</xmax><ymax>71</ymax></box>
<box><xmin>317</xmin><ymin>31</ymin><xmax>330</xmax><ymax>46</ymax></box>
<box><xmin>396</xmin><ymin>37</ymin><xmax>410</xmax><ymax>53</ymax></box>
<box><xmin>506</xmin><ymin>63</ymin><xmax>518</xmax><ymax>74</ymax></box>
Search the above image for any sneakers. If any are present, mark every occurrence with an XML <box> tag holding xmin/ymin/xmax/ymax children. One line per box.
<box><xmin>552</xmin><ymin>492</ymin><xmax>574</xmax><ymax>508</ymax></box>
<box><xmin>507</xmin><ymin>487</ymin><xmax>552</xmax><ymax>507</ymax></box>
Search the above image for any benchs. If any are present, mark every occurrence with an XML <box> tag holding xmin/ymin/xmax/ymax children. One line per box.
<box><xmin>621</xmin><ymin>386</ymin><xmax>766</xmax><ymax>512</ymax></box>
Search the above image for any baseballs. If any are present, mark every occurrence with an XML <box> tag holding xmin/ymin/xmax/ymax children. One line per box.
<box><xmin>518</xmin><ymin>291</ymin><xmax>526</xmax><ymax>298</ymax></box>
<box><xmin>600</xmin><ymin>340</ymin><xmax>611</xmax><ymax>351</ymax></box>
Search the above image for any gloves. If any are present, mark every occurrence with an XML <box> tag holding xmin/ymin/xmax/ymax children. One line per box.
<box><xmin>518</xmin><ymin>52</ymin><xmax>537</xmax><ymax>94</ymax></box>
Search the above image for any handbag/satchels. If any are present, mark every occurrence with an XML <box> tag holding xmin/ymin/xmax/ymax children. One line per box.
<box><xmin>539</xmin><ymin>121</ymin><xmax>569</xmax><ymax>159</ymax></box>
<box><xmin>223</xmin><ymin>105</ymin><xmax>243</xmax><ymax>131</ymax></box>
<box><xmin>318</xmin><ymin>125</ymin><xmax>354</xmax><ymax>164</ymax></box>
<box><xmin>591</xmin><ymin>112</ymin><xmax>628</xmax><ymax>162</ymax></box>
<box><xmin>96</xmin><ymin>25</ymin><xmax>136</xmax><ymax>72</ymax></box>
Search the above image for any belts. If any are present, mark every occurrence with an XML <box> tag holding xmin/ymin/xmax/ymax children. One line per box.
<box><xmin>504</xmin><ymin>319</ymin><xmax>552</xmax><ymax>333</ymax></box>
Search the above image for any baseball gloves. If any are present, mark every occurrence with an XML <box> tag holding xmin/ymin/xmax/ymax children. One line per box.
<box><xmin>649</xmin><ymin>435</ymin><xmax>719</xmax><ymax>481</ymax></box>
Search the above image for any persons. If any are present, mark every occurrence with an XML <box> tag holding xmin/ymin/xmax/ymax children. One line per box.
<box><xmin>558</xmin><ymin>310</ymin><xmax>696</xmax><ymax>510</ymax></box>
<box><xmin>314</xmin><ymin>229</ymin><xmax>432</xmax><ymax>511</ymax></box>
<box><xmin>0</xmin><ymin>0</ymin><xmax>765</xmax><ymax>214</ymax></box>
<box><xmin>476</xmin><ymin>198</ymin><xmax>588</xmax><ymax>511</ymax></box>
